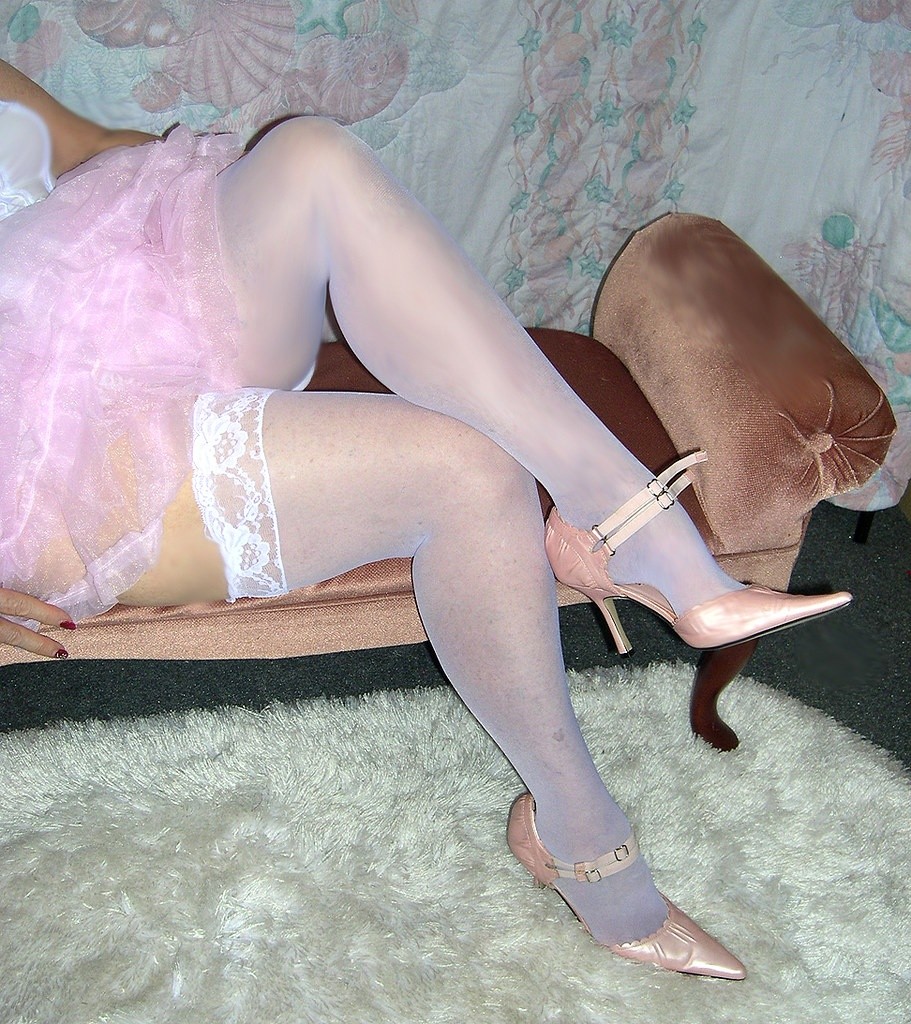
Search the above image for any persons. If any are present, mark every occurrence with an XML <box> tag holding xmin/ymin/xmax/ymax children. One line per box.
<box><xmin>0</xmin><ymin>61</ymin><xmax>853</xmax><ymax>981</ymax></box>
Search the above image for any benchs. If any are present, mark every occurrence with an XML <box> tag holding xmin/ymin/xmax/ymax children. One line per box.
<box><xmin>0</xmin><ymin>213</ymin><xmax>893</xmax><ymax>753</ymax></box>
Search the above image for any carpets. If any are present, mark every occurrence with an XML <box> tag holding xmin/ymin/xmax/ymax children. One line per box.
<box><xmin>0</xmin><ymin>662</ymin><xmax>911</xmax><ymax>1024</ymax></box>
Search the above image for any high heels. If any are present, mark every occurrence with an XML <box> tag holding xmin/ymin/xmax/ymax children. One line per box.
<box><xmin>543</xmin><ymin>450</ymin><xmax>854</xmax><ymax>654</ymax></box>
<box><xmin>504</xmin><ymin>793</ymin><xmax>747</xmax><ymax>981</ymax></box>
